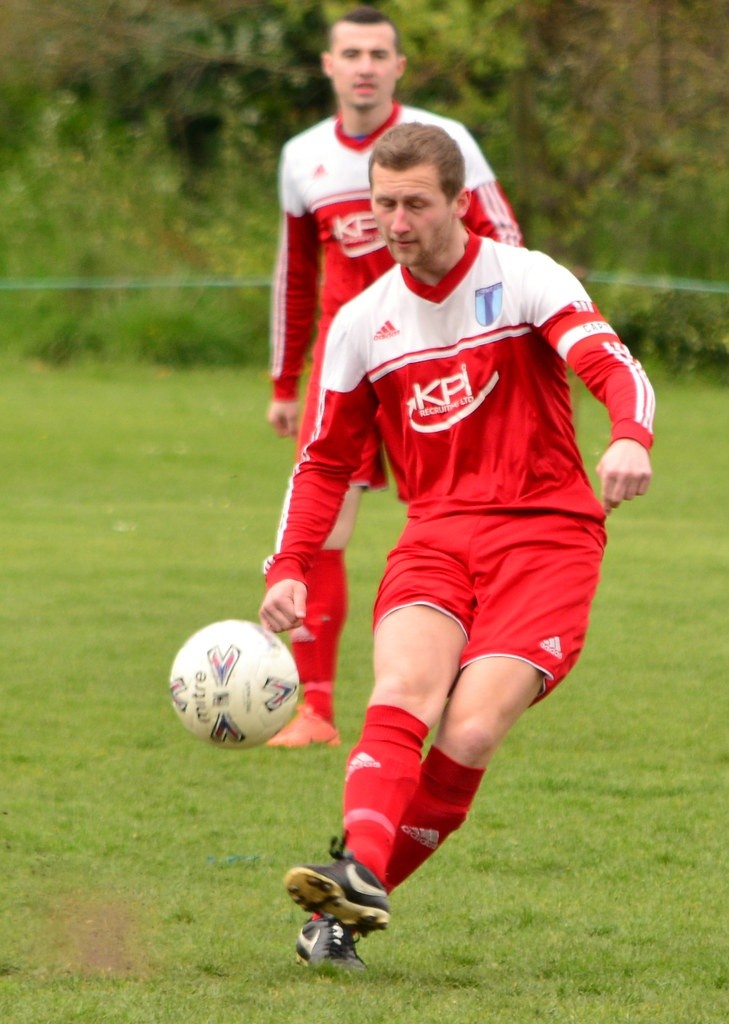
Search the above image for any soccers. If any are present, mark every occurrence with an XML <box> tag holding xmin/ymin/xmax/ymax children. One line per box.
<box><xmin>168</xmin><ymin>617</ymin><xmax>303</xmax><ymax>751</ymax></box>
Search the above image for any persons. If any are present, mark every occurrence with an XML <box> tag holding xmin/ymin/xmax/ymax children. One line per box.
<box><xmin>259</xmin><ymin>125</ymin><xmax>656</xmax><ymax>972</ymax></box>
<box><xmin>266</xmin><ymin>10</ymin><xmax>524</xmax><ymax>748</ymax></box>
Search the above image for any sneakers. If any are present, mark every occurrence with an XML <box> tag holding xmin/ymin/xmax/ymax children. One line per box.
<box><xmin>285</xmin><ymin>832</ymin><xmax>392</xmax><ymax>931</ymax></box>
<box><xmin>266</xmin><ymin>711</ymin><xmax>341</xmax><ymax>753</ymax></box>
<box><xmin>292</xmin><ymin>909</ymin><xmax>372</xmax><ymax>981</ymax></box>
